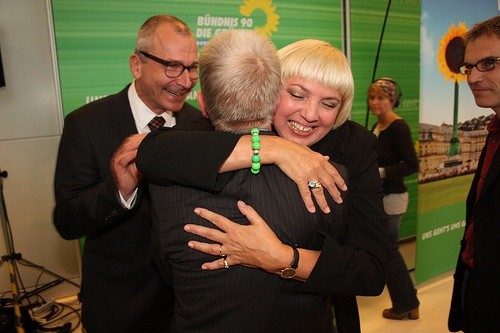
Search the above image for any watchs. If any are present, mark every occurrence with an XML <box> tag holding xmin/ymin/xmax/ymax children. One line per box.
<box><xmin>278</xmin><ymin>244</ymin><xmax>300</xmax><ymax>279</ymax></box>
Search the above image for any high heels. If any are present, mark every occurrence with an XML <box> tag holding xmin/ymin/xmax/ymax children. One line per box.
<box><xmin>383</xmin><ymin>307</ymin><xmax>418</xmax><ymax>319</ymax></box>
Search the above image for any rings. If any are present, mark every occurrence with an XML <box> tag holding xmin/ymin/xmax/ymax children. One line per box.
<box><xmin>220</xmin><ymin>246</ymin><xmax>225</xmax><ymax>257</ymax></box>
<box><xmin>309</xmin><ymin>181</ymin><xmax>322</xmax><ymax>188</ymax></box>
<box><xmin>223</xmin><ymin>257</ymin><xmax>229</xmax><ymax>269</ymax></box>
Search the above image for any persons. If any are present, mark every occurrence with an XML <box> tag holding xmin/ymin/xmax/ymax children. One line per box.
<box><xmin>53</xmin><ymin>13</ymin><xmax>221</xmax><ymax>333</ymax></box>
<box><xmin>136</xmin><ymin>39</ymin><xmax>388</xmax><ymax>333</ymax></box>
<box><xmin>447</xmin><ymin>16</ymin><xmax>500</xmax><ymax>333</ymax></box>
<box><xmin>366</xmin><ymin>77</ymin><xmax>419</xmax><ymax>320</ymax></box>
<box><xmin>149</xmin><ymin>29</ymin><xmax>350</xmax><ymax>333</ymax></box>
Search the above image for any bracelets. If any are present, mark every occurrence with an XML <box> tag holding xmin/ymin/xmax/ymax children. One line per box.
<box><xmin>250</xmin><ymin>128</ymin><xmax>261</xmax><ymax>174</ymax></box>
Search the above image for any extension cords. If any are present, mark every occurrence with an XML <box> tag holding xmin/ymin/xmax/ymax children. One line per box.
<box><xmin>32</xmin><ymin>298</ymin><xmax>56</xmax><ymax>314</ymax></box>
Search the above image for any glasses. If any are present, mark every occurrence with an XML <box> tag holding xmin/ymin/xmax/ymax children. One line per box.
<box><xmin>457</xmin><ymin>56</ymin><xmax>500</xmax><ymax>75</ymax></box>
<box><xmin>136</xmin><ymin>50</ymin><xmax>200</xmax><ymax>79</ymax></box>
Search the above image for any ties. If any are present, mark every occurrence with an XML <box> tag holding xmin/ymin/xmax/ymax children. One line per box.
<box><xmin>147</xmin><ymin>116</ymin><xmax>166</xmax><ymax>131</ymax></box>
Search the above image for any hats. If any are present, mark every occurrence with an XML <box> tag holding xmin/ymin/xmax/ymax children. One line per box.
<box><xmin>371</xmin><ymin>77</ymin><xmax>401</xmax><ymax>106</ymax></box>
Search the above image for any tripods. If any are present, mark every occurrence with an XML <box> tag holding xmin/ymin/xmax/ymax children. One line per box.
<box><xmin>0</xmin><ymin>168</ymin><xmax>82</xmax><ymax>333</ymax></box>
